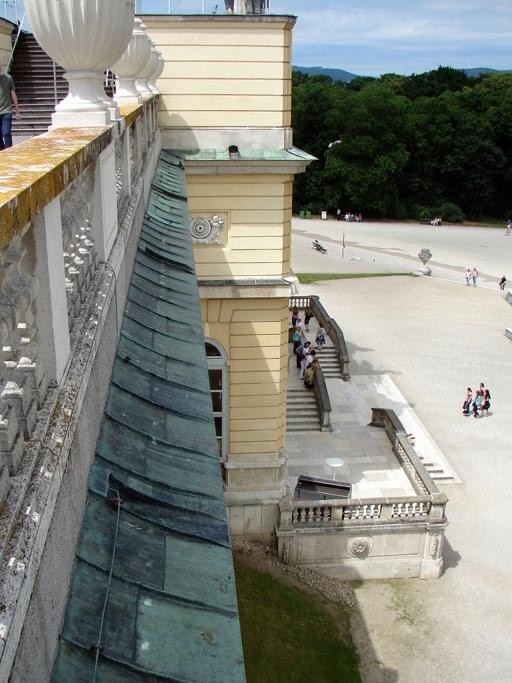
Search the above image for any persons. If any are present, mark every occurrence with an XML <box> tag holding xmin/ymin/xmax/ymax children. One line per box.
<box><xmin>431</xmin><ymin>217</ymin><xmax>443</xmax><ymax>226</ymax></box>
<box><xmin>506</xmin><ymin>218</ymin><xmax>512</xmax><ymax>233</ymax></box>
<box><xmin>225</xmin><ymin>0</ymin><xmax>234</xmax><ymax>15</ymax></box>
<box><xmin>465</xmin><ymin>269</ymin><xmax>471</xmax><ymax>285</ymax></box>
<box><xmin>0</xmin><ymin>61</ymin><xmax>21</xmax><ymax>150</ymax></box>
<box><xmin>499</xmin><ymin>275</ymin><xmax>507</xmax><ymax>290</ymax></box>
<box><xmin>463</xmin><ymin>383</ymin><xmax>491</xmax><ymax>419</ymax></box>
<box><xmin>473</xmin><ymin>268</ymin><xmax>479</xmax><ymax>287</ymax></box>
<box><xmin>291</xmin><ymin>305</ymin><xmax>326</xmax><ymax>390</ymax></box>
<box><xmin>337</xmin><ymin>208</ymin><xmax>363</xmax><ymax>222</ymax></box>
